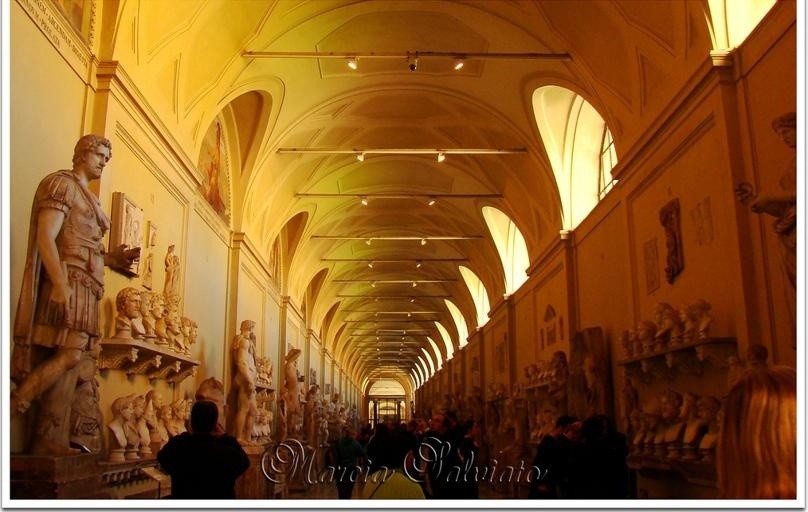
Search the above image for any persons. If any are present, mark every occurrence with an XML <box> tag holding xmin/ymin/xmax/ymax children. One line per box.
<box><xmin>415</xmin><ymin>352</ymin><xmax>567</xmax><ymax>439</ymax></box>
<box><xmin>157</xmin><ymin>401</ymin><xmax>249</xmax><ymax>499</ymax></box>
<box><xmin>735</xmin><ymin>113</ymin><xmax>796</xmax><ymax>350</ymax></box>
<box><xmin>11</xmin><ymin>135</ymin><xmax>140</xmax><ymax>456</ymax></box>
<box><xmin>105</xmin><ymin>245</ymin><xmax>365</xmax><ymax>436</ymax></box>
<box><xmin>529</xmin><ymin>415</ymin><xmax>636</xmax><ymax>499</ymax></box>
<box><xmin>324</xmin><ymin>412</ymin><xmax>485</xmax><ymax>499</ymax></box>
<box><xmin>715</xmin><ymin>364</ymin><xmax>796</xmax><ymax>499</ymax></box>
<box><xmin>617</xmin><ymin>299</ymin><xmax>767</xmax><ymax>450</ymax></box>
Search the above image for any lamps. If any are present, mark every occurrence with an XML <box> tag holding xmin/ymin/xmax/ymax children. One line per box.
<box><xmin>347</xmin><ymin>55</ymin><xmax>464</xmax><ymax>72</ymax></box>
<box><xmin>360</xmin><ymin>196</ymin><xmax>434</xmax><ymax>206</ymax></box>
<box><xmin>365</xmin><ymin>237</ymin><xmax>426</xmax><ymax>245</ymax></box>
<box><xmin>368</xmin><ymin>260</ymin><xmax>421</xmax><ymax>268</ymax></box>
<box><xmin>356</xmin><ymin>151</ymin><xmax>446</xmax><ymax>162</ymax></box>
<box><xmin>370</xmin><ymin>280</ymin><xmax>417</xmax><ymax>288</ymax></box>
